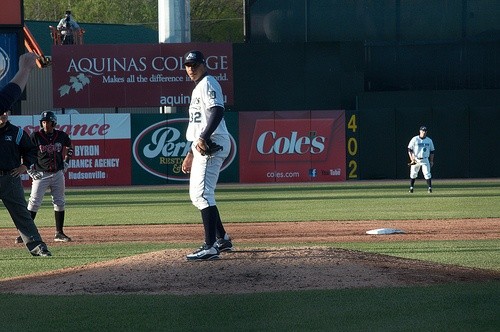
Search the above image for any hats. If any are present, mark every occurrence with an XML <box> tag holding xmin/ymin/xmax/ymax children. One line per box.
<box><xmin>183</xmin><ymin>50</ymin><xmax>205</xmax><ymax>65</ymax></box>
<box><xmin>420</xmin><ymin>126</ymin><xmax>427</xmax><ymax>130</ymax></box>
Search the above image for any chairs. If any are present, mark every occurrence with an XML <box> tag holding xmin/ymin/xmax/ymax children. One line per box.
<box><xmin>49</xmin><ymin>23</ymin><xmax>86</xmax><ymax>46</ymax></box>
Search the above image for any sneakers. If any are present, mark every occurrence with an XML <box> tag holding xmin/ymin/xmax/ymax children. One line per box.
<box><xmin>214</xmin><ymin>237</ymin><xmax>232</xmax><ymax>252</ymax></box>
<box><xmin>15</xmin><ymin>236</ymin><xmax>22</xmax><ymax>242</ymax></box>
<box><xmin>53</xmin><ymin>232</ymin><xmax>70</xmax><ymax>241</ymax></box>
<box><xmin>186</xmin><ymin>242</ymin><xmax>218</xmax><ymax>261</ymax></box>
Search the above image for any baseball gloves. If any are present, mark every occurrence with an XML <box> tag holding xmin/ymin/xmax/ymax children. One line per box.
<box><xmin>195</xmin><ymin>140</ymin><xmax>223</xmax><ymax>156</ymax></box>
<box><xmin>408</xmin><ymin>159</ymin><xmax>416</xmax><ymax>166</ymax></box>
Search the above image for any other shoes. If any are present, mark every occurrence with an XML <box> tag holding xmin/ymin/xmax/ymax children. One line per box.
<box><xmin>409</xmin><ymin>189</ymin><xmax>413</xmax><ymax>193</ymax></box>
<box><xmin>428</xmin><ymin>188</ymin><xmax>432</xmax><ymax>193</ymax></box>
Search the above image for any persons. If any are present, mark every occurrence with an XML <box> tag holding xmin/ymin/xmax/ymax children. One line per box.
<box><xmin>0</xmin><ymin>110</ymin><xmax>52</xmax><ymax>257</ymax></box>
<box><xmin>407</xmin><ymin>125</ymin><xmax>435</xmax><ymax>194</ymax></box>
<box><xmin>0</xmin><ymin>52</ymin><xmax>37</xmax><ymax>116</ymax></box>
<box><xmin>57</xmin><ymin>10</ymin><xmax>82</xmax><ymax>46</ymax></box>
<box><xmin>181</xmin><ymin>50</ymin><xmax>233</xmax><ymax>261</ymax></box>
<box><xmin>15</xmin><ymin>111</ymin><xmax>74</xmax><ymax>243</ymax></box>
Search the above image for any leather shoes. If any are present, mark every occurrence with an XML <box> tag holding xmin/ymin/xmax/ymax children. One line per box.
<box><xmin>38</xmin><ymin>250</ymin><xmax>51</xmax><ymax>256</ymax></box>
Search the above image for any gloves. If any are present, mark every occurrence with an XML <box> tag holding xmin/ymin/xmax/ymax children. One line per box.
<box><xmin>64</xmin><ymin>162</ymin><xmax>68</xmax><ymax>172</ymax></box>
<box><xmin>205</xmin><ymin>140</ymin><xmax>223</xmax><ymax>155</ymax></box>
<box><xmin>27</xmin><ymin>169</ymin><xmax>42</xmax><ymax>179</ymax></box>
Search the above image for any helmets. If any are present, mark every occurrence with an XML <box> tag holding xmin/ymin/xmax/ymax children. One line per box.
<box><xmin>39</xmin><ymin>111</ymin><xmax>57</xmax><ymax>127</ymax></box>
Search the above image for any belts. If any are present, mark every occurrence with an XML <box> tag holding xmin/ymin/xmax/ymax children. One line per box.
<box><xmin>417</xmin><ymin>158</ymin><xmax>424</xmax><ymax>159</ymax></box>
